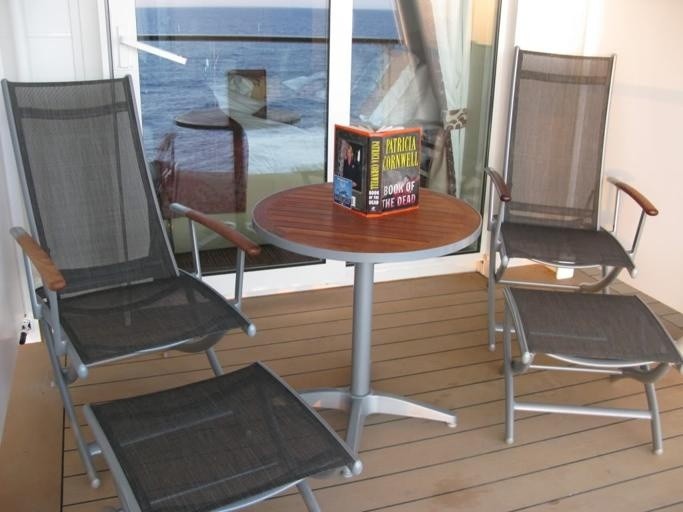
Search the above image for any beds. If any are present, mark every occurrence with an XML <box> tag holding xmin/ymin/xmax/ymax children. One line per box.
<box><xmin>138</xmin><ymin>47</ymin><xmax>440</xmax><ymax>255</ymax></box>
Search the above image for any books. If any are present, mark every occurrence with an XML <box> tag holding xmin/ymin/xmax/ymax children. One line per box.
<box><xmin>229</xmin><ymin>69</ymin><xmax>267</xmax><ymax>120</ymax></box>
<box><xmin>332</xmin><ymin>124</ymin><xmax>422</xmax><ymax>218</ymax></box>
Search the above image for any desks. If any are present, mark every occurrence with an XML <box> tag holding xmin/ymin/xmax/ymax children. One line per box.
<box><xmin>174</xmin><ymin>106</ymin><xmax>303</xmax><ymax>248</ymax></box>
<box><xmin>252</xmin><ymin>179</ymin><xmax>482</xmax><ymax>479</ymax></box>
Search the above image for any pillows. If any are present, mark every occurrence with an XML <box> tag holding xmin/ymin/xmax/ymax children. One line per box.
<box><xmin>299</xmin><ymin>47</ymin><xmax>432</xmax><ymax>129</ymax></box>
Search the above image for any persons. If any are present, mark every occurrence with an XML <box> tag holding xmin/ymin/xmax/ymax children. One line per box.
<box><xmin>343</xmin><ymin>145</ymin><xmax>360</xmax><ymax>190</ymax></box>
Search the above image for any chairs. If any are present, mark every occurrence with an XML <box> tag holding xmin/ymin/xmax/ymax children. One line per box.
<box><xmin>152</xmin><ymin>133</ymin><xmax>176</xmax><ymax>253</ymax></box>
<box><xmin>483</xmin><ymin>46</ymin><xmax>683</xmax><ymax>454</ymax></box>
<box><xmin>0</xmin><ymin>72</ymin><xmax>363</xmax><ymax>512</ymax></box>
